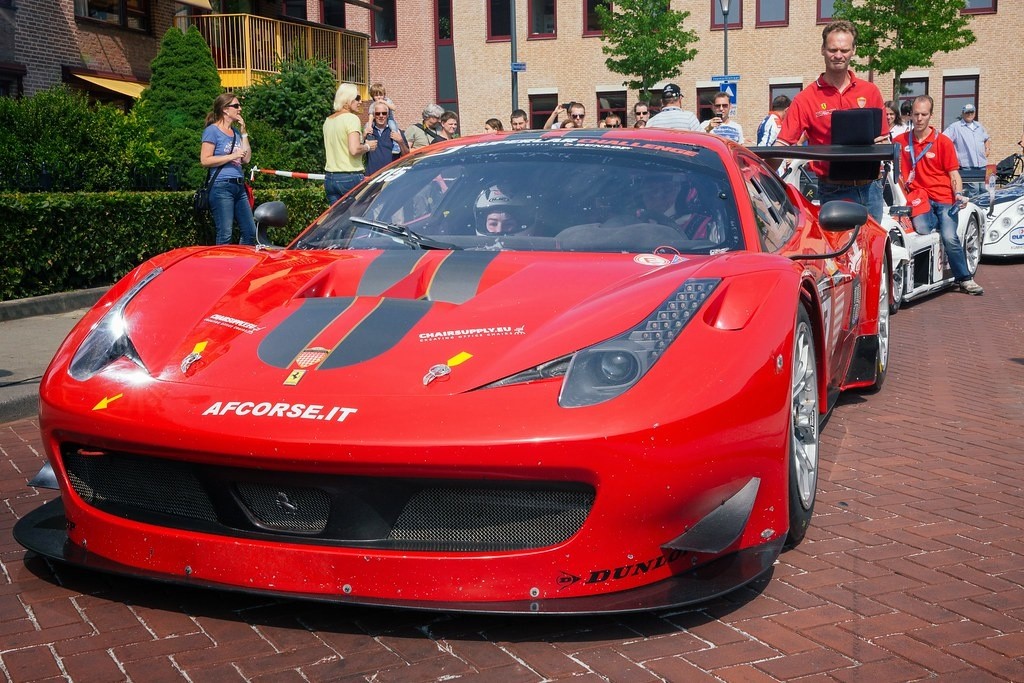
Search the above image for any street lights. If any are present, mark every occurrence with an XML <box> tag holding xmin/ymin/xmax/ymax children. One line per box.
<box><xmin>719</xmin><ymin>0</ymin><xmax>731</xmax><ymax>75</ymax></box>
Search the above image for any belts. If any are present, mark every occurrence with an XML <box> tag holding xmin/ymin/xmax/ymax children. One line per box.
<box><xmin>818</xmin><ymin>173</ymin><xmax>883</xmax><ymax>186</ymax></box>
<box><xmin>223</xmin><ymin>177</ymin><xmax>244</xmax><ymax>184</ymax></box>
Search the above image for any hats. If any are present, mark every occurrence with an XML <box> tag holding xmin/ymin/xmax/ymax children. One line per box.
<box><xmin>962</xmin><ymin>104</ymin><xmax>976</xmax><ymax>113</ymax></box>
<box><xmin>643</xmin><ymin>170</ymin><xmax>687</xmax><ymax>182</ymax></box>
<box><xmin>662</xmin><ymin>83</ymin><xmax>683</xmax><ymax>97</ymax></box>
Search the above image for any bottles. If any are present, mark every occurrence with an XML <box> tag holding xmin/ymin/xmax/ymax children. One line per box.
<box><xmin>989</xmin><ymin>173</ymin><xmax>995</xmax><ymax>199</ymax></box>
<box><xmin>948</xmin><ymin>198</ymin><xmax>966</xmax><ymax>216</ymax></box>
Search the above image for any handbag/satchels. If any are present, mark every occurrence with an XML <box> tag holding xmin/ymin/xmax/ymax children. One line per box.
<box><xmin>193</xmin><ymin>187</ymin><xmax>210</xmax><ymax>210</ymax></box>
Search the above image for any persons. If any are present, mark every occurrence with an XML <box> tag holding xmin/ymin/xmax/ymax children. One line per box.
<box><xmin>885</xmin><ymin>96</ymin><xmax>990</xmax><ymax>295</ymax></box>
<box><xmin>763</xmin><ymin>20</ymin><xmax>889</xmax><ymax>226</ymax></box>
<box><xmin>323</xmin><ymin>83</ymin><xmax>378</xmax><ymax>208</ymax></box>
<box><xmin>363</xmin><ymin>83</ymin><xmax>809</xmax><ymax>228</ymax></box>
<box><xmin>628</xmin><ymin>166</ymin><xmax>720</xmax><ymax>244</ymax></box>
<box><xmin>201</xmin><ymin>93</ymin><xmax>256</xmax><ymax>246</ymax></box>
<box><xmin>472</xmin><ymin>181</ymin><xmax>538</xmax><ymax>237</ymax></box>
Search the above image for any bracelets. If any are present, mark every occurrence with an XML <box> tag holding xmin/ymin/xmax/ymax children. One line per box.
<box><xmin>365</xmin><ymin>143</ymin><xmax>370</xmax><ymax>152</ymax></box>
<box><xmin>241</xmin><ymin>133</ymin><xmax>248</xmax><ymax>137</ymax></box>
<box><xmin>954</xmin><ymin>192</ymin><xmax>962</xmax><ymax>196</ymax></box>
<box><xmin>986</xmin><ymin>147</ymin><xmax>990</xmax><ymax>150</ymax></box>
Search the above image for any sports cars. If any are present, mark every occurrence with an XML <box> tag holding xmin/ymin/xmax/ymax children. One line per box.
<box><xmin>37</xmin><ymin>127</ymin><xmax>890</xmax><ymax>614</ymax></box>
<box><xmin>970</xmin><ymin>171</ymin><xmax>1024</xmax><ymax>257</ymax></box>
<box><xmin>777</xmin><ymin>154</ymin><xmax>985</xmax><ymax>314</ymax></box>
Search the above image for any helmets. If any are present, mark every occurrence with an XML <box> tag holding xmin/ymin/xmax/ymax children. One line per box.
<box><xmin>473</xmin><ymin>178</ymin><xmax>537</xmax><ymax>236</ymax></box>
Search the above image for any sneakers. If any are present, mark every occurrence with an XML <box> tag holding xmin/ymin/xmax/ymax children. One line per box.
<box><xmin>959</xmin><ymin>277</ymin><xmax>983</xmax><ymax>295</ymax></box>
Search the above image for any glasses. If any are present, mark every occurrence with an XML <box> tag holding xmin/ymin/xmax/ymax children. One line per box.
<box><xmin>604</xmin><ymin>123</ymin><xmax>621</xmax><ymax>128</ymax></box>
<box><xmin>374</xmin><ymin>111</ymin><xmax>389</xmax><ymax>115</ymax></box>
<box><xmin>355</xmin><ymin>94</ymin><xmax>361</xmax><ymax>101</ymax></box>
<box><xmin>570</xmin><ymin>114</ymin><xmax>585</xmax><ymax>118</ymax></box>
<box><xmin>635</xmin><ymin>111</ymin><xmax>649</xmax><ymax>115</ymax></box>
<box><xmin>645</xmin><ymin>182</ymin><xmax>679</xmax><ymax>195</ymax></box>
<box><xmin>225</xmin><ymin>103</ymin><xmax>241</xmax><ymax>109</ymax></box>
<box><xmin>714</xmin><ymin>103</ymin><xmax>730</xmax><ymax>107</ymax></box>
